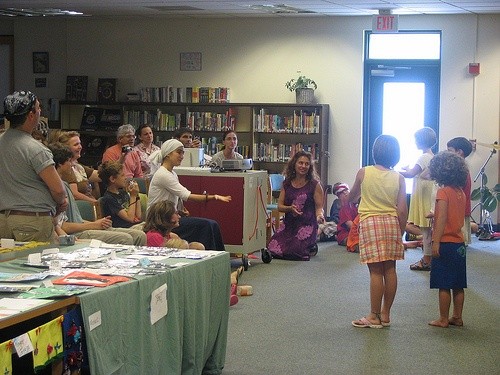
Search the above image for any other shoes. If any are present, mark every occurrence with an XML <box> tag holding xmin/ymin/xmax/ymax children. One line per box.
<box><xmin>309</xmin><ymin>243</ymin><xmax>318</xmax><ymax>256</ymax></box>
<box><xmin>410</xmin><ymin>259</ymin><xmax>431</xmax><ymax>271</ymax></box>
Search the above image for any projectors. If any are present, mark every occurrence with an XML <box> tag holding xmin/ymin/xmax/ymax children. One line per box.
<box><xmin>222</xmin><ymin>158</ymin><xmax>253</xmax><ymax>170</ymax></box>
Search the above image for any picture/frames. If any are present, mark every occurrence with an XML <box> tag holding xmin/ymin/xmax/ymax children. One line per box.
<box><xmin>32</xmin><ymin>52</ymin><xmax>49</xmax><ymax>73</ymax></box>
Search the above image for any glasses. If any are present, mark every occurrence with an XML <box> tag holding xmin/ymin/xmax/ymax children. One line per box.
<box><xmin>125</xmin><ymin>135</ymin><xmax>137</xmax><ymax>139</ymax></box>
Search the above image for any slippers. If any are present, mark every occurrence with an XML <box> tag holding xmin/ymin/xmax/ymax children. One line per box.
<box><xmin>352</xmin><ymin>316</ymin><xmax>391</xmax><ymax>329</ymax></box>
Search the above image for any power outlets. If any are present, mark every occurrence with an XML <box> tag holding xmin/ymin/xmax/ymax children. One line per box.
<box><xmin>325</xmin><ymin>185</ymin><xmax>332</xmax><ymax>194</ymax></box>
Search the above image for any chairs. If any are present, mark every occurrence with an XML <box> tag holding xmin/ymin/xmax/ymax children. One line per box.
<box><xmin>266</xmin><ymin>174</ymin><xmax>286</xmax><ymax>231</ymax></box>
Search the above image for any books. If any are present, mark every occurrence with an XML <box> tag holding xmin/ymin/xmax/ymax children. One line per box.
<box><xmin>123</xmin><ymin>86</ymin><xmax>320</xmax><ymax>164</ymax></box>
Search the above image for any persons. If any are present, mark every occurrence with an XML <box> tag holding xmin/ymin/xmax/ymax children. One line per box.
<box><xmin>0</xmin><ymin>90</ymin><xmax>473</xmax><ymax>327</ymax></box>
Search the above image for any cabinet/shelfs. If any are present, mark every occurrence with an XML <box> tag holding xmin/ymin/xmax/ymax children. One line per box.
<box><xmin>171</xmin><ymin>167</ymin><xmax>268</xmax><ymax>254</ymax></box>
<box><xmin>59</xmin><ymin>100</ymin><xmax>329</xmax><ymax>222</ymax></box>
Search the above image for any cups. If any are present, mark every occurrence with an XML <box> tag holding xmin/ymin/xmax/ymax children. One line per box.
<box><xmin>237</xmin><ymin>286</ymin><xmax>253</xmax><ymax>296</ymax></box>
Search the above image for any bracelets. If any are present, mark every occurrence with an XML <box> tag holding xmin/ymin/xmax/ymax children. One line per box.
<box><xmin>136</xmin><ymin>196</ymin><xmax>140</xmax><ymax>200</ymax></box>
<box><xmin>206</xmin><ymin>194</ymin><xmax>209</xmax><ymax>202</ymax></box>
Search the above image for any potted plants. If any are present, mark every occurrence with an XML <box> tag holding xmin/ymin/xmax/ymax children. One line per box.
<box><xmin>284</xmin><ymin>75</ymin><xmax>317</xmax><ymax>104</ymax></box>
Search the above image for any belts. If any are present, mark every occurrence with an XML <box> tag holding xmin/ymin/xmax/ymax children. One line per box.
<box><xmin>0</xmin><ymin>209</ymin><xmax>52</xmax><ymax>216</ymax></box>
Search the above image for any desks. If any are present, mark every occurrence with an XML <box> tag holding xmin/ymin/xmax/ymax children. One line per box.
<box><xmin>0</xmin><ymin>239</ymin><xmax>231</xmax><ymax>375</ymax></box>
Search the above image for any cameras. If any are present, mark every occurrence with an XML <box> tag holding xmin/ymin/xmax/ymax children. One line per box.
<box><xmin>122</xmin><ymin>145</ymin><xmax>131</xmax><ymax>152</ymax></box>
<box><xmin>59</xmin><ymin>234</ymin><xmax>75</xmax><ymax>245</ymax></box>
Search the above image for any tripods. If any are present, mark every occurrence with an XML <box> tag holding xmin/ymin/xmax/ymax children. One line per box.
<box><xmin>473</xmin><ymin>148</ymin><xmax>497</xmax><ymax>239</ymax></box>
